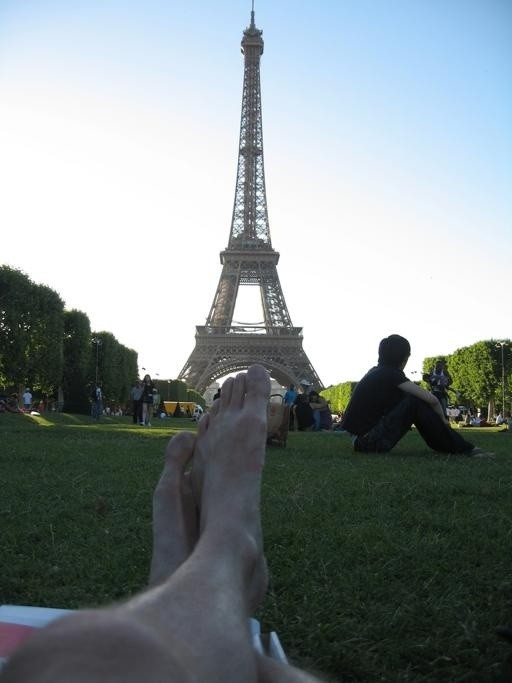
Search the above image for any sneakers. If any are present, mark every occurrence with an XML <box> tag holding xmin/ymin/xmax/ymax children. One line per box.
<box><xmin>134</xmin><ymin>421</ymin><xmax>151</xmax><ymax>427</ymax></box>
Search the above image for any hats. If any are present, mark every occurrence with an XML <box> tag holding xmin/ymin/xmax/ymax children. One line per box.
<box><xmin>299</xmin><ymin>380</ymin><xmax>311</xmax><ymax>386</ymax></box>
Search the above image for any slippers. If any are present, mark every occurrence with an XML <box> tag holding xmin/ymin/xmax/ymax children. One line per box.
<box><xmin>471</xmin><ymin>448</ymin><xmax>496</xmax><ymax>460</ymax></box>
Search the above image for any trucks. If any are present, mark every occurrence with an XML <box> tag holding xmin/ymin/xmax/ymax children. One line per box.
<box><xmin>158</xmin><ymin>401</ymin><xmax>205</xmax><ymax>418</ymax></box>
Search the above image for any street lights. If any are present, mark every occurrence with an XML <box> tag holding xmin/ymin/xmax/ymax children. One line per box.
<box><xmin>494</xmin><ymin>340</ymin><xmax>509</xmax><ymax>419</ymax></box>
<box><xmin>92</xmin><ymin>336</ymin><xmax>103</xmax><ymax>387</ymax></box>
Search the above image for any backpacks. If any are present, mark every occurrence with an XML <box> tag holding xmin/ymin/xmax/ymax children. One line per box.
<box><xmin>91</xmin><ymin>391</ymin><xmax>96</xmax><ymax>398</ymax></box>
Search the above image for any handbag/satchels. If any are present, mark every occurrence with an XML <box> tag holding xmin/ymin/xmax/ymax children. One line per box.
<box><xmin>265</xmin><ymin>393</ymin><xmax>290</xmax><ymax>448</ymax></box>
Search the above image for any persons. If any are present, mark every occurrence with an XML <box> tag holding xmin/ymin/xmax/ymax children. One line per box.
<box><xmin>448</xmin><ymin>399</ymin><xmax>512</xmax><ymax>432</ymax></box>
<box><xmin>1</xmin><ymin>365</ymin><xmax>335</xmax><ymax>682</ymax></box>
<box><xmin>343</xmin><ymin>334</ymin><xmax>495</xmax><ymax>461</ymax></box>
<box><xmin>1</xmin><ymin>372</ymin><xmax>221</xmax><ymax>427</ymax></box>
<box><xmin>422</xmin><ymin>360</ymin><xmax>453</xmax><ymax>419</ymax></box>
<box><xmin>282</xmin><ymin>379</ymin><xmax>342</xmax><ymax>432</ymax></box>
